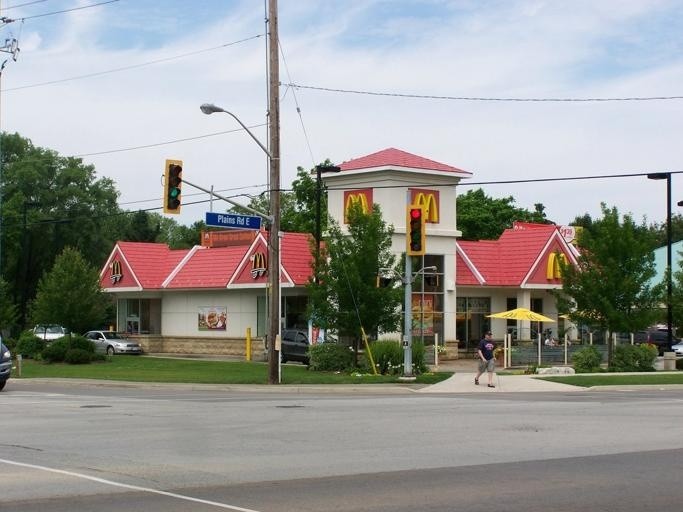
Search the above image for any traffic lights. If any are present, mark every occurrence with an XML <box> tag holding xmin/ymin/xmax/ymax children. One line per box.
<box><xmin>409</xmin><ymin>208</ymin><xmax>421</xmax><ymax>251</ymax></box>
<box><xmin>163</xmin><ymin>159</ymin><xmax>184</xmax><ymax>215</ymax></box>
<box><xmin>425</xmin><ymin>276</ymin><xmax>437</xmax><ymax>286</ymax></box>
<box><xmin>375</xmin><ymin>276</ymin><xmax>391</xmax><ymax>288</ymax></box>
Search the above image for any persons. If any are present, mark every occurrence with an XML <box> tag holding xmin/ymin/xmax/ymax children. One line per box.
<box><xmin>473</xmin><ymin>329</ymin><xmax>497</xmax><ymax>388</ymax></box>
<box><xmin>545</xmin><ymin>335</ymin><xmax>558</xmax><ymax>347</ymax></box>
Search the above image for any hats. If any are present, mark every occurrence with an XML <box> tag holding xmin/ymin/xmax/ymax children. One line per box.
<box><xmin>483</xmin><ymin>330</ymin><xmax>493</xmax><ymax>335</ymax></box>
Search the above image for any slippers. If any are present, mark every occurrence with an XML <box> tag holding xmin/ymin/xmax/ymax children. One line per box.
<box><xmin>488</xmin><ymin>384</ymin><xmax>495</xmax><ymax>388</ymax></box>
<box><xmin>475</xmin><ymin>377</ymin><xmax>479</xmax><ymax>385</ymax></box>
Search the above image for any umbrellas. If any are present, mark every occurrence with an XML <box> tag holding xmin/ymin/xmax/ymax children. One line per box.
<box><xmin>578</xmin><ymin>309</ymin><xmax>604</xmax><ymax>319</ymax></box>
<box><xmin>484</xmin><ymin>306</ymin><xmax>556</xmax><ymax>323</ymax></box>
<box><xmin>558</xmin><ymin>312</ymin><xmax>581</xmax><ymax>319</ymax></box>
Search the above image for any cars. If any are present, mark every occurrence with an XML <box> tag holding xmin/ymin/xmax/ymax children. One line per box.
<box><xmin>0</xmin><ymin>340</ymin><xmax>13</xmax><ymax>391</ymax></box>
<box><xmin>578</xmin><ymin>323</ymin><xmax>601</xmax><ymax>344</ymax></box>
<box><xmin>30</xmin><ymin>324</ymin><xmax>144</xmax><ymax>355</ymax></box>
<box><xmin>616</xmin><ymin>330</ymin><xmax>683</xmax><ymax>356</ymax></box>
<box><xmin>281</xmin><ymin>329</ymin><xmax>309</xmax><ymax>365</ymax></box>
<box><xmin>486</xmin><ymin>326</ymin><xmax>538</xmax><ymax>342</ymax></box>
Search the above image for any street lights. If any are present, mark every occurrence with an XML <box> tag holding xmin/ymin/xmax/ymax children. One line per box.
<box><xmin>647</xmin><ymin>171</ymin><xmax>672</xmax><ymax>351</ymax></box>
<box><xmin>199</xmin><ymin>101</ymin><xmax>281</xmax><ymax>382</ymax></box>
